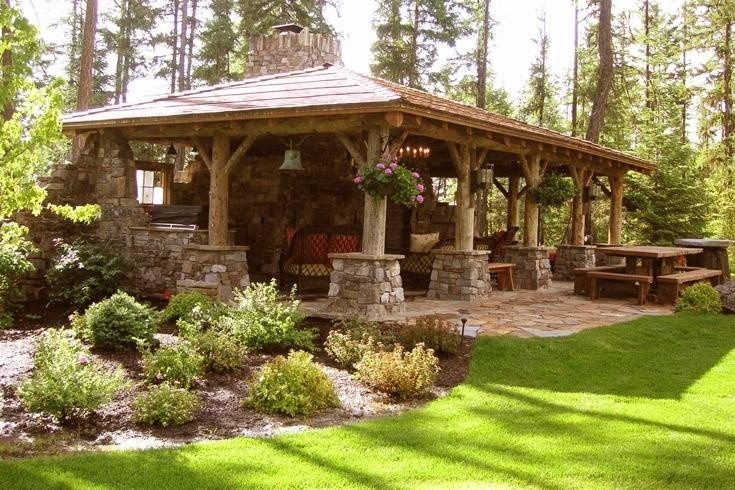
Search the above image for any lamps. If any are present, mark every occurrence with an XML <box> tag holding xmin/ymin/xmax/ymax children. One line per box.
<box><xmin>278</xmin><ymin>134</ymin><xmax>314</xmax><ymax>172</ymax></box>
<box><xmin>471</xmin><ymin>162</ymin><xmax>497</xmax><ymax>191</ymax></box>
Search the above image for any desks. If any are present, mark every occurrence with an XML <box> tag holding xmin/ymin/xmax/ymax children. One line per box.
<box><xmin>593</xmin><ymin>245</ymin><xmax>703</xmax><ymax>304</ymax></box>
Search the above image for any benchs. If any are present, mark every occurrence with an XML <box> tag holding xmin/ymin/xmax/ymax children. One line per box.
<box><xmin>279</xmin><ymin>222</ymin><xmax>363</xmax><ymax>295</ymax></box>
<box><xmin>571</xmin><ymin>262</ymin><xmax>723</xmax><ymax>307</ymax></box>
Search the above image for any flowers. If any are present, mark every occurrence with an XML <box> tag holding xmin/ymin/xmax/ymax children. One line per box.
<box><xmin>353</xmin><ymin>146</ymin><xmax>427</xmax><ymax>211</ymax></box>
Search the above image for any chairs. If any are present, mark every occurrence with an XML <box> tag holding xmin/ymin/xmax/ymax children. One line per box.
<box><xmin>385</xmin><ymin>226</ymin><xmax>521</xmax><ymax>294</ymax></box>
